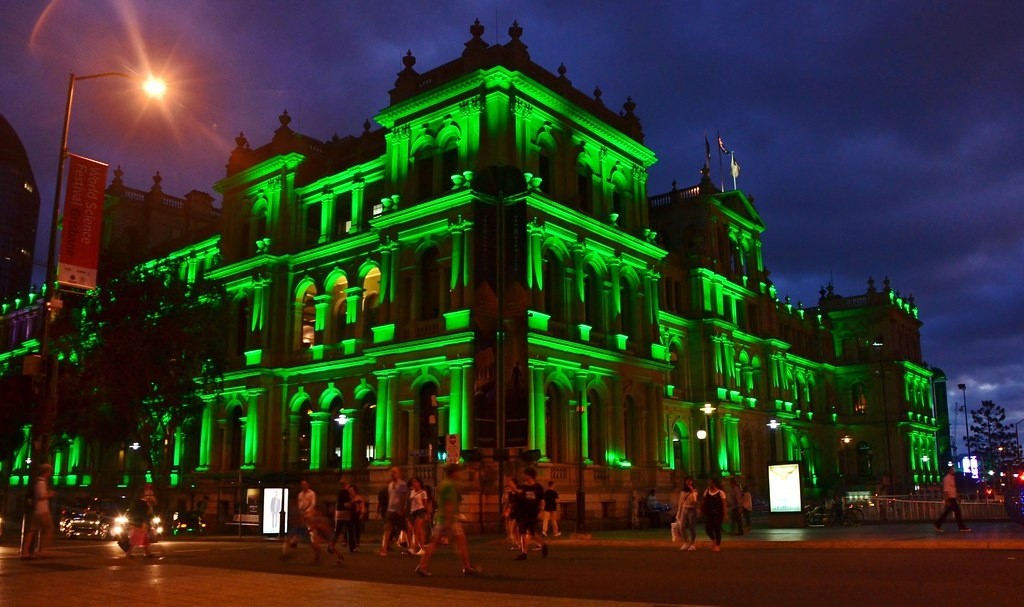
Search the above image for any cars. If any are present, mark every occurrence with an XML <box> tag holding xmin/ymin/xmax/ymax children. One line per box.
<box><xmin>21</xmin><ymin>491</ymin><xmax>208</xmax><ymax>541</ymax></box>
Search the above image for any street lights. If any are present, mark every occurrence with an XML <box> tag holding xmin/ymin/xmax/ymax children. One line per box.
<box><xmin>873</xmin><ymin>337</ymin><xmax>895</xmax><ymax>495</ymax></box>
<box><xmin>957</xmin><ymin>383</ymin><xmax>971</xmax><ymax>458</ymax></box>
<box><xmin>38</xmin><ymin>62</ymin><xmax>185</xmax><ymax>352</ymax></box>
<box><xmin>698</xmin><ymin>403</ymin><xmax>717</xmax><ymax>477</ymax></box>
<box><xmin>696</xmin><ymin>430</ymin><xmax>709</xmax><ymax>479</ymax></box>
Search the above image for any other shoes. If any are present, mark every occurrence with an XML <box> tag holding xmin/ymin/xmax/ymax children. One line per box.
<box><xmin>415</xmin><ymin>566</ymin><xmax>432</xmax><ymax>577</ymax></box>
<box><xmin>125</xmin><ymin>554</ymin><xmax>134</xmax><ymax>559</ymax></box>
<box><xmin>554</xmin><ymin>532</ymin><xmax>561</xmax><ymax>536</ymax></box>
<box><xmin>688</xmin><ymin>545</ymin><xmax>695</xmax><ymax>551</ymax></box>
<box><xmin>542</xmin><ymin>531</ymin><xmax>547</xmax><ymax>537</ymax></box>
<box><xmin>712</xmin><ymin>545</ymin><xmax>721</xmax><ymax>552</ymax></box>
<box><xmin>960</xmin><ymin>527</ymin><xmax>972</xmax><ymax>531</ymax></box>
<box><xmin>326</xmin><ymin>537</ymin><xmax>430</xmax><ymax>558</ymax></box>
<box><xmin>681</xmin><ymin>543</ymin><xmax>689</xmax><ymax>550</ymax></box>
<box><xmin>464</xmin><ymin>567</ymin><xmax>479</xmax><ymax>576</ymax></box>
<box><xmin>932</xmin><ymin>523</ymin><xmax>944</xmax><ymax>532</ymax></box>
<box><xmin>144</xmin><ymin>554</ymin><xmax>155</xmax><ymax>558</ymax></box>
<box><xmin>505</xmin><ymin>534</ymin><xmax>548</xmax><ymax>561</ymax></box>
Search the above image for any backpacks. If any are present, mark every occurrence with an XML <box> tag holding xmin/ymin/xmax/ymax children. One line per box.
<box><xmin>22</xmin><ymin>478</ymin><xmax>46</xmax><ymax>513</ymax></box>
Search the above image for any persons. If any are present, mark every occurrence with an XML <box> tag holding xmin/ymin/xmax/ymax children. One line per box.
<box><xmin>197</xmin><ymin>496</ymin><xmax>209</xmax><ymax>513</ymax></box>
<box><xmin>933</xmin><ymin>467</ymin><xmax>972</xmax><ymax>532</ymax></box>
<box><xmin>23</xmin><ymin>463</ymin><xmax>56</xmax><ymax>556</ymax></box>
<box><xmin>728</xmin><ymin>479</ymin><xmax>744</xmax><ymax>536</ymax></box>
<box><xmin>290</xmin><ymin>480</ymin><xmax>324</xmax><ymax>565</ymax></box>
<box><xmin>742</xmin><ymin>486</ymin><xmax>752</xmax><ymax>531</ymax></box>
<box><xmin>502</xmin><ymin>466</ymin><xmax>548</xmax><ymax>561</ymax></box>
<box><xmin>126</xmin><ymin>487</ymin><xmax>154</xmax><ymax>558</ymax></box>
<box><xmin>699</xmin><ymin>477</ymin><xmax>727</xmax><ymax>552</ymax></box>
<box><xmin>415</xmin><ymin>462</ymin><xmax>480</xmax><ymax>576</ymax></box>
<box><xmin>327</xmin><ymin>466</ymin><xmax>436</xmax><ymax>557</ymax></box>
<box><xmin>542</xmin><ymin>481</ymin><xmax>561</xmax><ymax>537</ymax></box>
<box><xmin>646</xmin><ymin>489</ymin><xmax>656</xmax><ymax>508</ymax></box>
<box><xmin>675</xmin><ymin>475</ymin><xmax>699</xmax><ymax>550</ymax></box>
<box><xmin>271</xmin><ymin>490</ymin><xmax>280</xmax><ymax>528</ymax></box>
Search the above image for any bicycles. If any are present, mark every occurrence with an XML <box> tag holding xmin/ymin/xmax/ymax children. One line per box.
<box><xmin>804</xmin><ymin>500</ymin><xmax>865</xmax><ymax>528</ymax></box>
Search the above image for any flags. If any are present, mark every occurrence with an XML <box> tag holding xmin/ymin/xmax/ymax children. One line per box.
<box><xmin>718</xmin><ymin>134</ymin><xmax>731</xmax><ymax>154</ymax></box>
<box><xmin>731</xmin><ymin>152</ymin><xmax>739</xmax><ymax>177</ymax></box>
<box><xmin>704</xmin><ymin>133</ymin><xmax>710</xmax><ymax>159</ymax></box>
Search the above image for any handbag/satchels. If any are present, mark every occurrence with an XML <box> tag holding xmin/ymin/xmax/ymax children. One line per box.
<box><xmin>671</xmin><ymin>519</ymin><xmax>681</xmax><ymax>543</ymax></box>
<box><xmin>118</xmin><ymin>534</ymin><xmax>131</xmax><ymax>552</ymax></box>
